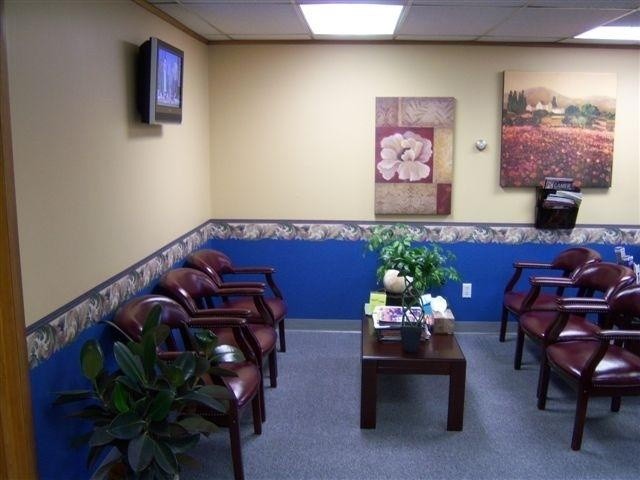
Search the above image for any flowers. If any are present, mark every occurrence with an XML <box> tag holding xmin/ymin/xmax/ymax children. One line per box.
<box><xmin>377</xmin><ymin>130</ymin><xmax>434</xmax><ymax>184</ymax></box>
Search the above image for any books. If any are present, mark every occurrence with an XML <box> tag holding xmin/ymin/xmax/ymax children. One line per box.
<box><xmin>372</xmin><ymin>305</ymin><xmax>432</xmax><ymax>343</ymax></box>
<box><xmin>543</xmin><ymin>177</ymin><xmax>583</xmax><ymax>209</ymax></box>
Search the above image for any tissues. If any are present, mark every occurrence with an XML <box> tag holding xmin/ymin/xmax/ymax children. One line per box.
<box><xmin>431</xmin><ymin>295</ymin><xmax>455</xmax><ymax>335</ymax></box>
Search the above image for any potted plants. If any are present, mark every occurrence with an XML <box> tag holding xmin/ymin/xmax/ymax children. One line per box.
<box><xmin>366</xmin><ymin>222</ymin><xmax>464</xmax><ymax>353</ymax></box>
<box><xmin>51</xmin><ymin>304</ymin><xmax>245</xmax><ymax>480</ymax></box>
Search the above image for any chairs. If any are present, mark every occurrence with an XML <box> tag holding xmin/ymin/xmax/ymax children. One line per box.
<box><xmin>115</xmin><ymin>294</ymin><xmax>265</xmax><ymax>480</ymax></box>
<box><xmin>185</xmin><ymin>247</ymin><xmax>287</xmax><ymax>380</ymax></box>
<box><xmin>153</xmin><ymin>266</ymin><xmax>279</xmax><ymax>425</ymax></box>
<box><xmin>500</xmin><ymin>247</ymin><xmax>640</xmax><ymax>451</ymax></box>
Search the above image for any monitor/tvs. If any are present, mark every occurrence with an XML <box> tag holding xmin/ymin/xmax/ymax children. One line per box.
<box><xmin>139</xmin><ymin>36</ymin><xmax>185</xmax><ymax>126</ymax></box>
